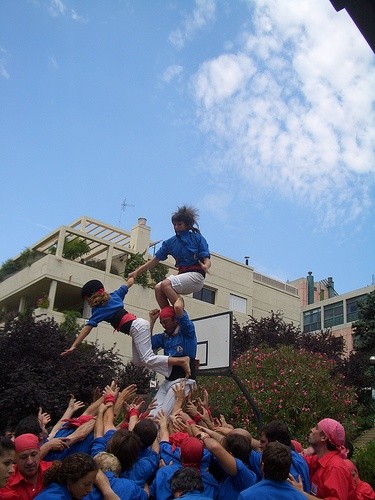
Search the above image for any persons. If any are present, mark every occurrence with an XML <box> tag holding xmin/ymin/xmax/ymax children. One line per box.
<box><xmin>58</xmin><ymin>279</ymin><xmax>193</xmax><ymax>378</ymax></box>
<box><xmin>149</xmin><ymin>297</ymin><xmax>198</xmax><ymax>419</ymax></box>
<box><xmin>126</xmin><ymin>205</ymin><xmax>212</xmax><ymax>311</ymax></box>
<box><xmin>0</xmin><ymin>382</ymin><xmax>374</xmax><ymax>500</ymax></box>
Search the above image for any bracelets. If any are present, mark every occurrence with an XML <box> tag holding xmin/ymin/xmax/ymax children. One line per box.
<box><xmin>187</xmin><ymin>420</ymin><xmax>196</xmax><ymax>425</ymax></box>
<box><xmin>139</xmin><ymin>413</ymin><xmax>149</xmax><ymax>419</ymax></box>
<box><xmin>206</xmin><ymin>408</ymin><xmax>211</xmax><ymax>417</ymax></box>
<box><xmin>193</xmin><ymin>413</ymin><xmax>201</xmax><ymax>421</ymax></box>
<box><xmin>104</xmin><ymin>395</ymin><xmax>117</xmax><ymax>405</ymax></box>
<box><xmin>120</xmin><ymin>422</ymin><xmax>128</xmax><ymax>429</ymax></box>
<box><xmin>128</xmin><ymin>409</ymin><xmax>138</xmax><ymax>417</ymax></box>
